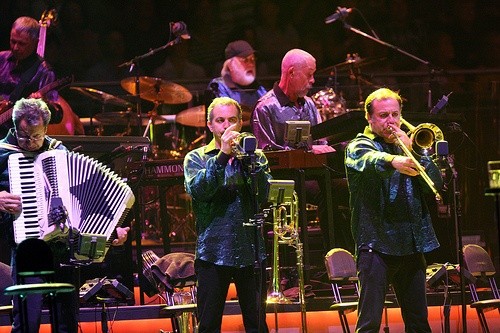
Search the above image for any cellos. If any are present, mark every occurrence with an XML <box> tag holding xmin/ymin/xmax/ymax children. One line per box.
<box><xmin>37</xmin><ymin>10</ymin><xmax>75</xmax><ymax>136</ymax></box>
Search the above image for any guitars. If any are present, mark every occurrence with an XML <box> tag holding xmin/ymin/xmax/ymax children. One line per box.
<box><xmin>0</xmin><ymin>73</ymin><xmax>73</xmax><ymax>140</ymax></box>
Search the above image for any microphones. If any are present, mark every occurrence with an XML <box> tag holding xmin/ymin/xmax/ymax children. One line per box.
<box><xmin>325</xmin><ymin>7</ymin><xmax>352</xmax><ymax>23</ymax></box>
<box><xmin>171</xmin><ymin>21</ymin><xmax>188</xmax><ymax>35</ymax></box>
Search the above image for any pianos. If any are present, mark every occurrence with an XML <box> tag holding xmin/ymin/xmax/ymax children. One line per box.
<box><xmin>139</xmin><ymin>147</ymin><xmax>327</xmax><ymax>180</ymax></box>
<box><xmin>310</xmin><ymin>111</ymin><xmax>464</xmax><ymax>179</ymax></box>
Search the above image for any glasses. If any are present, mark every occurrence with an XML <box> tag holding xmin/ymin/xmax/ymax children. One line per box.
<box><xmin>15</xmin><ymin>127</ymin><xmax>45</xmax><ymax>142</ymax></box>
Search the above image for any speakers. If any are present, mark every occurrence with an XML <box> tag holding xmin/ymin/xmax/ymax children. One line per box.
<box><xmin>484</xmin><ymin>190</ymin><xmax>500</xmax><ymax>286</ymax></box>
<box><xmin>56</xmin><ymin>249</ymin><xmax>133</xmax><ymax>288</ymax></box>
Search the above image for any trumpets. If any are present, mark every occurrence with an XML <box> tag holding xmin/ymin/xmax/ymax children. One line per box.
<box><xmin>230</xmin><ymin>132</ymin><xmax>255</xmax><ymax>159</ymax></box>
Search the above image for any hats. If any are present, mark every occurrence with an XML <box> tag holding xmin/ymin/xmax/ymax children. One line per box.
<box><xmin>224</xmin><ymin>40</ymin><xmax>256</xmax><ymax>60</ymax></box>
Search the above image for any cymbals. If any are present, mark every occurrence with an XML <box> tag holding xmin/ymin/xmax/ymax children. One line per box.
<box><xmin>68</xmin><ymin>86</ymin><xmax>136</xmax><ymax>108</ymax></box>
<box><xmin>175</xmin><ymin>104</ymin><xmax>257</xmax><ymax>128</ymax></box>
<box><xmin>120</xmin><ymin>76</ymin><xmax>193</xmax><ymax>104</ymax></box>
<box><xmin>318</xmin><ymin>55</ymin><xmax>388</xmax><ymax>74</ymax></box>
<box><xmin>92</xmin><ymin>111</ymin><xmax>168</xmax><ymax>127</ymax></box>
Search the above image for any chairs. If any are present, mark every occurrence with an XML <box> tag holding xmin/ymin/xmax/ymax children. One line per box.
<box><xmin>459</xmin><ymin>244</ymin><xmax>500</xmax><ymax>333</ymax></box>
<box><xmin>143</xmin><ymin>251</ymin><xmax>202</xmax><ymax>333</ymax></box>
<box><xmin>325</xmin><ymin>248</ymin><xmax>393</xmax><ymax>333</ymax></box>
<box><xmin>3</xmin><ymin>238</ymin><xmax>76</xmax><ymax>333</ymax></box>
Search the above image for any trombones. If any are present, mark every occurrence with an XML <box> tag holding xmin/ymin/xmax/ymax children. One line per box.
<box><xmin>386</xmin><ymin>115</ymin><xmax>444</xmax><ymax>201</ymax></box>
<box><xmin>272</xmin><ymin>189</ymin><xmax>307</xmax><ymax>333</ymax></box>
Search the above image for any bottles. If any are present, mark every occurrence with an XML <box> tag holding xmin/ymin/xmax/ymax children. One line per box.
<box><xmin>173</xmin><ymin>284</ymin><xmax>191</xmax><ymax>304</ymax></box>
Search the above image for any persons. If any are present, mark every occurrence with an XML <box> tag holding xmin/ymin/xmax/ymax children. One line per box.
<box><xmin>345</xmin><ymin>88</ymin><xmax>443</xmax><ymax>333</ymax></box>
<box><xmin>0</xmin><ymin>0</ymin><xmax>500</xmax><ymax>89</ymax></box>
<box><xmin>0</xmin><ymin>98</ymin><xmax>130</xmax><ymax>333</ymax></box>
<box><xmin>208</xmin><ymin>42</ymin><xmax>269</xmax><ymax>132</ymax></box>
<box><xmin>184</xmin><ymin>98</ymin><xmax>272</xmax><ymax>333</ymax></box>
<box><xmin>0</xmin><ymin>16</ymin><xmax>61</xmax><ymax>139</ymax></box>
<box><xmin>253</xmin><ymin>48</ymin><xmax>352</xmax><ymax>298</ymax></box>
